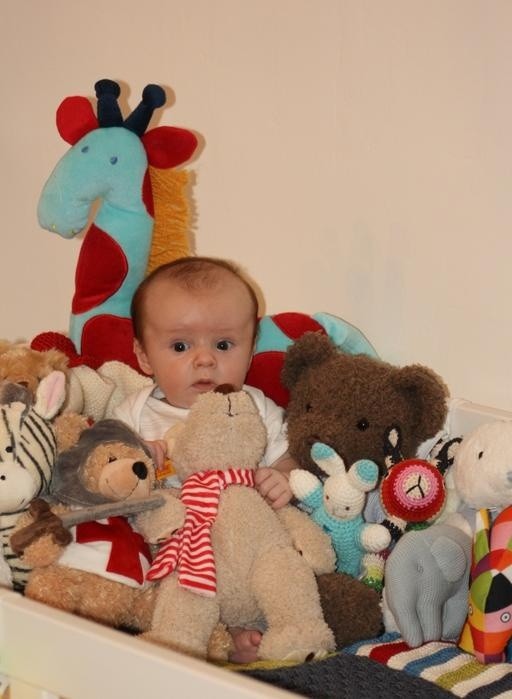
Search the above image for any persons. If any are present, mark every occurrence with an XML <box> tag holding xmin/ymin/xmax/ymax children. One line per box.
<box><xmin>111</xmin><ymin>257</ymin><xmax>302</xmax><ymax>666</ymax></box>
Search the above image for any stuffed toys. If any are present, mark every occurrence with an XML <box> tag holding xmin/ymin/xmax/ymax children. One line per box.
<box><xmin>7</xmin><ymin>418</ymin><xmax>162</xmax><ymax>633</ymax></box>
<box><xmin>434</xmin><ymin>414</ymin><xmax>512</xmax><ymax>525</ymax></box>
<box><xmin>0</xmin><ymin>370</ymin><xmax>66</xmax><ymax>598</ymax></box>
<box><xmin>0</xmin><ymin>339</ymin><xmax>68</xmax><ymax>400</ymax></box>
<box><xmin>458</xmin><ymin>507</ymin><xmax>512</xmax><ymax>665</ymax></box>
<box><xmin>279</xmin><ymin>331</ymin><xmax>450</xmax><ymax>651</ymax></box>
<box><xmin>134</xmin><ymin>383</ymin><xmax>337</xmax><ymax>664</ymax></box>
<box><xmin>289</xmin><ymin>441</ymin><xmax>393</xmax><ymax>578</ymax></box>
<box><xmin>381</xmin><ymin>522</ymin><xmax>473</xmax><ymax>648</ymax></box>
<box><xmin>360</xmin><ymin>424</ymin><xmax>463</xmax><ymax>589</ymax></box>
<box><xmin>30</xmin><ymin>78</ymin><xmax>382</xmax><ymax>411</ymax></box>
